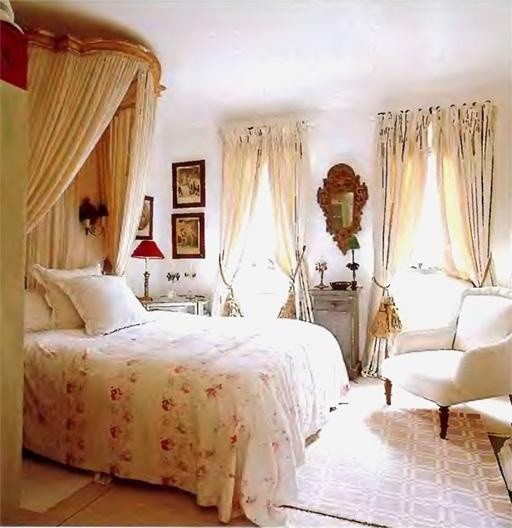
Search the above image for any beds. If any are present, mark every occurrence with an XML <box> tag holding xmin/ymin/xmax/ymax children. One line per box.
<box><xmin>22</xmin><ymin>311</ymin><xmax>349</xmax><ymax>527</ymax></box>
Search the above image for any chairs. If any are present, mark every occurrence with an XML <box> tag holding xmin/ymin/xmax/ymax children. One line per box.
<box><xmin>382</xmin><ymin>287</ymin><xmax>512</xmax><ymax>439</ymax></box>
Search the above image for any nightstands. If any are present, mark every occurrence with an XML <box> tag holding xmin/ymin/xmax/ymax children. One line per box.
<box><xmin>146</xmin><ymin>295</ymin><xmax>209</xmax><ymax>317</ymax></box>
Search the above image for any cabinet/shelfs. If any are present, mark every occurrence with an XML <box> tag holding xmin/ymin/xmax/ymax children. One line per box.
<box><xmin>308</xmin><ymin>286</ymin><xmax>361</xmax><ymax>380</ymax></box>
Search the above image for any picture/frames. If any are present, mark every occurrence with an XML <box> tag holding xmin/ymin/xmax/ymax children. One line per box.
<box><xmin>172</xmin><ymin>160</ymin><xmax>205</xmax><ymax>208</ymax></box>
<box><xmin>135</xmin><ymin>196</ymin><xmax>154</xmax><ymax>240</ymax></box>
<box><xmin>171</xmin><ymin>212</ymin><xmax>205</xmax><ymax>259</ymax></box>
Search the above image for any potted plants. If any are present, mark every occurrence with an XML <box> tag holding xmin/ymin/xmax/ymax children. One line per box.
<box><xmin>166</xmin><ymin>272</ymin><xmax>196</xmax><ymax>300</ymax></box>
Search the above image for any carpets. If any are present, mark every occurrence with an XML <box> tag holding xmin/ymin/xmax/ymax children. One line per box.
<box><xmin>18</xmin><ymin>456</ymin><xmax>116</xmax><ymax>526</ymax></box>
<box><xmin>273</xmin><ymin>374</ymin><xmax>512</xmax><ymax>528</ymax></box>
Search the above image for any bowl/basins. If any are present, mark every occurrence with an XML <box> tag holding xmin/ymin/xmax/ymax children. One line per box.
<box><xmin>329</xmin><ymin>281</ymin><xmax>351</xmax><ymax>290</ymax></box>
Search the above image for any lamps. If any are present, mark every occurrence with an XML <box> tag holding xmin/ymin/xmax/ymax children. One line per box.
<box><xmin>131</xmin><ymin>240</ymin><xmax>164</xmax><ymax>301</ymax></box>
<box><xmin>79</xmin><ymin>197</ymin><xmax>108</xmax><ymax>238</ymax></box>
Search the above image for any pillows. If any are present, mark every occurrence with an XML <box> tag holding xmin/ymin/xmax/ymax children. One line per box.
<box><xmin>32</xmin><ymin>258</ymin><xmax>105</xmax><ymax>329</ymax></box>
<box><xmin>23</xmin><ymin>288</ymin><xmax>50</xmax><ymax>330</ymax></box>
<box><xmin>54</xmin><ymin>276</ymin><xmax>147</xmax><ymax>334</ymax></box>
